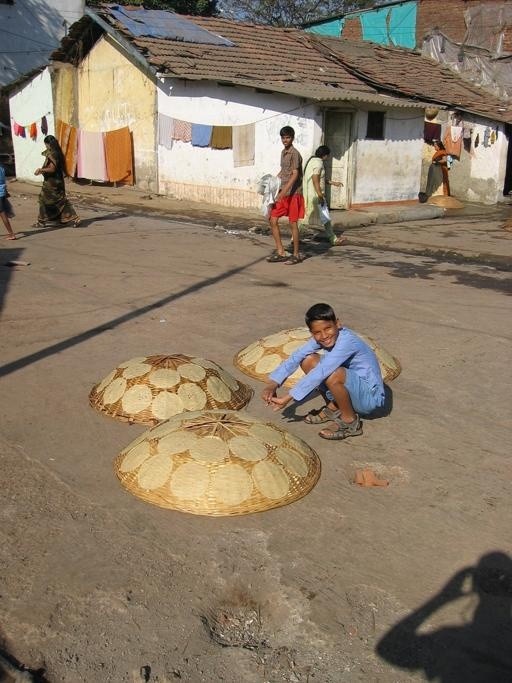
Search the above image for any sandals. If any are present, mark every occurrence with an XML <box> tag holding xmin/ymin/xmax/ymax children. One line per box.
<box><xmin>304</xmin><ymin>406</ymin><xmax>363</xmax><ymax>440</ymax></box>
<box><xmin>266</xmin><ymin>254</ymin><xmax>302</xmax><ymax>265</ymax></box>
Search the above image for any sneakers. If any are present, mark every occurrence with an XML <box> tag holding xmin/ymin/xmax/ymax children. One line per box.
<box><xmin>31</xmin><ymin>222</ymin><xmax>45</xmax><ymax>227</ymax></box>
<box><xmin>73</xmin><ymin>220</ymin><xmax>81</xmax><ymax>227</ymax></box>
<box><xmin>331</xmin><ymin>237</ymin><xmax>344</xmax><ymax>245</ymax></box>
<box><xmin>291</xmin><ymin>239</ymin><xmax>302</xmax><ymax>244</ymax></box>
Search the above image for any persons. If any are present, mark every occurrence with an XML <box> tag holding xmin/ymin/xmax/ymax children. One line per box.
<box><xmin>266</xmin><ymin>126</ymin><xmax>344</xmax><ymax>265</ymax></box>
<box><xmin>426</xmin><ymin>141</ymin><xmax>449</xmax><ymax>196</ymax></box>
<box><xmin>0</xmin><ymin>159</ymin><xmax>16</xmax><ymax>240</ymax></box>
<box><xmin>30</xmin><ymin>135</ymin><xmax>81</xmax><ymax>228</ymax></box>
<box><xmin>262</xmin><ymin>303</ymin><xmax>385</xmax><ymax>440</ymax></box>
